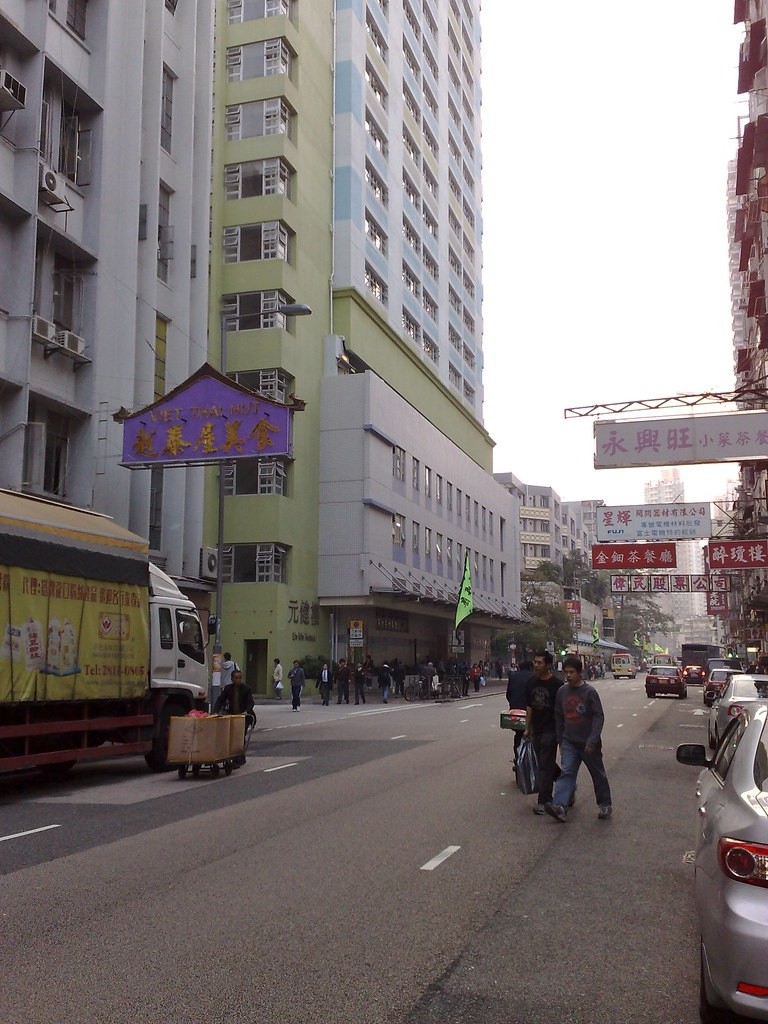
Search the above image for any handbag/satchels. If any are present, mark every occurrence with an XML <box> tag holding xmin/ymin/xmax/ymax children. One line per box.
<box><xmin>515</xmin><ymin>738</ymin><xmax>539</xmax><ymax>795</ymax></box>
<box><xmin>276</xmin><ymin>681</ymin><xmax>284</xmax><ymax>690</ymax></box>
<box><xmin>480</xmin><ymin>676</ymin><xmax>485</xmax><ymax>688</ymax></box>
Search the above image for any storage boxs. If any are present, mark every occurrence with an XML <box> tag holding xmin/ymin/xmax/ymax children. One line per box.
<box><xmin>168</xmin><ymin>714</ymin><xmax>245</xmax><ymax>764</ymax></box>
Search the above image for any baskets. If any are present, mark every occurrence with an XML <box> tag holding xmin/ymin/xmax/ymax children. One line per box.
<box><xmin>500</xmin><ymin>711</ymin><xmax>527</xmax><ymax>731</ymax></box>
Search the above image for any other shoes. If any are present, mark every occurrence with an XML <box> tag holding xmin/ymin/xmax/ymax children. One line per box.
<box><xmin>364</xmin><ymin>687</ymin><xmax>372</xmax><ymax>690</ymax></box>
<box><xmin>322</xmin><ymin>702</ymin><xmax>325</xmax><ymax>705</ymax></box>
<box><xmin>337</xmin><ymin>702</ymin><xmax>341</xmax><ymax>704</ymax></box>
<box><xmin>346</xmin><ymin>701</ymin><xmax>350</xmax><ymax>704</ymax></box>
<box><xmin>355</xmin><ymin>703</ymin><xmax>359</xmax><ymax>705</ymax></box>
<box><xmin>232</xmin><ymin>758</ymin><xmax>246</xmax><ymax>769</ymax></box>
<box><xmin>326</xmin><ymin>703</ymin><xmax>329</xmax><ymax>706</ymax></box>
<box><xmin>384</xmin><ymin>701</ymin><xmax>388</xmax><ymax>703</ymax></box>
<box><xmin>363</xmin><ymin>700</ymin><xmax>365</xmax><ymax>704</ymax></box>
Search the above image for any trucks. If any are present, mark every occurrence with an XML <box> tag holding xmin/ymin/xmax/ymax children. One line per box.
<box><xmin>682</xmin><ymin>644</ymin><xmax>721</xmax><ymax>667</ymax></box>
<box><xmin>0</xmin><ymin>489</ymin><xmax>219</xmax><ymax>785</ymax></box>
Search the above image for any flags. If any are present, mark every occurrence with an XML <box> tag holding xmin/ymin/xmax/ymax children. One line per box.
<box><xmin>591</xmin><ymin>615</ymin><xmax>600</xmax><ymax>650</ymax></box>
<box><xmin>632</xmin><ymin>631</ymin><xmax>664</xmax><ymax>655</ymax></box>
<box><xmin>454</xmin><ymin>548</ymin><xmax>474</xmax><ymax>648</ymax></box>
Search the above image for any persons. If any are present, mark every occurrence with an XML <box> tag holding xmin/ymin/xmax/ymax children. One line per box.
<box><xmin>352</xmin><ymin>654</ymin><xmax>536</xmax><ymax>706</ymax></box>
<box><xmin>220</xmin><ymin>652</ymin><xmax>241</xmax><ymax>715</ymax></box>
<box><xmin>523</xmin><ymin>650</ymin><xmax>577</xmax><ymax>815</ymax></box>
<box><xmin>271</xmin><ymin>658</ymin><xmax>283</xmax><ymax>700</ymax></box>
<box><xmin>288</xmin><ymin>660</ymin><xmax>305</xmax><ymax>712</ymax></box>
<box><xmin>316</xmin><ymin>663</ymin><xmax>333</xmax><ymax>706</ymax></box>
<box><xmin>584</xmin><ymin>659</ymin><xmax>611</xmax><ymax>681</ymax></box>
<box><xmin>505</xmin><ymin>661</ymin><xmax>535</xmax><ymax>772</ymax></box>
<box><xmin>741</xmin><ymin>661</ymin><xmax>768</xmax><ymax>675</ymax></box>
<box><xmin>544</xmin><ymin>657</ymin><xmax>613</xmax><ymax>823</ymax></box>
<box><xmin>213</xmin><ymin>656</ymin><xmax>222</xmax><ymax>670</ymax></box>
<box><xmin>210</xmin><ymin>670</ymin><xmax>258</xmax><ymax>749</ymax></box>
<box><xmin>334</xmin><ymin>658</ymin><xmax>352</xmax><ymax>705</ymax></box>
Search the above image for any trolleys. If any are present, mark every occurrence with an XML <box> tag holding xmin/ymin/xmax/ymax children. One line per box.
<box><xmin>169</xmin><ymin>710</ymin><xmax>257</xmax><ymax>777</ymax></box>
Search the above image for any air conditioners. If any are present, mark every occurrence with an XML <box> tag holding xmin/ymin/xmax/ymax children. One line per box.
<box><xmin>57</xmin><ymin>331</ymin><xmax>84</xmax><ymax>354</ymax></box>
<box><xmin>0</xmin><ymin>70</ymin><xmax>28</xmax><ymax>105</ymax></box>
<box><xmin>200</xmin><ymin>547</ymin><xmax>217</xmax><ymax>581</ymax></box>
<box><xmin>38</xmin><ymin>164</ymin><xmax>65</xmax><ymax>203</ymax></box>
<box><xmin>32</xmin><ymin>315</ymin><xmax>56</xmax><ymax>341</ymax></box>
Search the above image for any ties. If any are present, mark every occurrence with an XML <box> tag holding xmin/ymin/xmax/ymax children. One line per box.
<box><xmin>324</xmin><ymin>671</ymin><xmax>325</xmax><ymax>680</ymax></box>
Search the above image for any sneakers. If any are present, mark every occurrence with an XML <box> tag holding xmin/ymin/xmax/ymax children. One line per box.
<box><xmin>297</xmin><ymin>706</ymin><xmax>300</xmax><ymax>712</ymax></box>
<box><xmin>293</xmin><ymin>709</ymin><xmax>296</xmax><ymax>712</ymax></box>
<box><xmin>597</xmin><ymin>803</ymin><xmax>613</xmax><ymax>819</ymax></box>
<box><xmin>568</xmin><ymin>782</ymin><xmax>577</xmax><ymax>806</ymax></box>
<box><xmin>533</xmin><ymin>804</ymin><xmax>547</xmax><ymax>815</ymax></box>
<box><xmin>544</xmin><ymin>802</ymin><xmax>566</xmax><ymax>823</ymax></box>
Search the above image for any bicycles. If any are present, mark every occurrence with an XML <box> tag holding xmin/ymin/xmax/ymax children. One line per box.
<box><xmin>403</xmin><ymin>679</ymin><xmax>428</xmax><ymax>702</ymax></box>
<box><xmin>500</xmin><ymin>709</ymin><xmax>537</xmax><ymax>794</ymax></box>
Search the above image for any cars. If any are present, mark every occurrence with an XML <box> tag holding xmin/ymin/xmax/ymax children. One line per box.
<box><xmin>703</xmin><ymin>669</ymin><xmax>745</xmax><ymax>706</ymax></box>
<box><xmin>676</xmin><ymin>701</ymin><xmax>768</xmax><ymax>1024</ymax></box>
<box><xmin>645</xmin><ymin>665</ymin><xmax>687</xmax><ymax>699</ymax></box>
<box><xmin>706</xmin><ymin>659</ymin><xmax>745</xmax><ymax>686</ymax></box>
<box><xmin>611</xmin><ymin>654</ymin><xmax>636</xmax><ymax>678</ymax></box>
<box><xmin>709</xmin><ymin>675</ymin><xmax>767</xmax><ymax>747</ymax></box>
<box><xmin>683</xmin><ymin>665</ymin><xmax>704</xmax><ymax>684</ymax></box>
